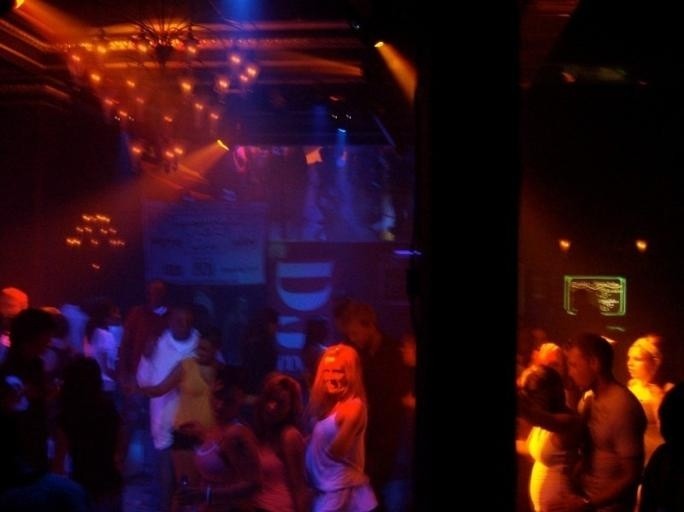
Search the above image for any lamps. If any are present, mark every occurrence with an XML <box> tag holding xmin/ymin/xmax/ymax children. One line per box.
<box><xmin>62</xmin><ymin>1</ymin><xmax>260</xmax><ymax>175</ymax></box>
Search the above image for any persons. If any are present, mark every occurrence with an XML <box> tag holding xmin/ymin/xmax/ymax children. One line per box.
<box><xmin>0</xmin><ymin>282</ymin><xmax>416</xmax><ymax>512</ymax></box>
<box><xmin>514</xmin><ymin>326</ymin><xmax>684</xmax><ymax>512</ymax></box>
